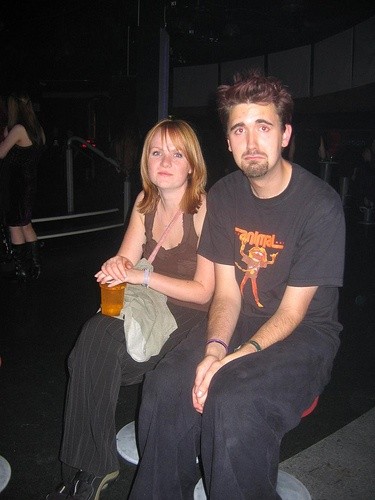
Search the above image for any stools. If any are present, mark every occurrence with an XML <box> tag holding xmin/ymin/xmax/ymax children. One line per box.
<box><xmin>193</xmin><ymin>394</ymin><xmax>320</xmax><ymax>500</ymax></box>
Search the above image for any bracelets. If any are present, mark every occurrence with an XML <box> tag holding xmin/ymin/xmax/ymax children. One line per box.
<box><xmin>141</xmin><ymin>268</ymin><xmax>151</xmax><ymax>289</ymax></box>
<box><xmin>247</xmin><ymin>338</ymin><xmax>261</xmax><ymax>353</ymax></box>
<box><xmin>206</xmin><ymin>338</ymin><xmax>229</xmax><ymax>356</ymax></box>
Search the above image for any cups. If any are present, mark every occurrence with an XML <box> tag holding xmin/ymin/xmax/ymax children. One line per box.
<box><xmin>100</xmin><ymin>281</ymin><xmax>127</xmax><ymax>317</ymax></box>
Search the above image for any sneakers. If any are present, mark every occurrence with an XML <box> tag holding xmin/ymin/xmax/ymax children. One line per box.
<box><xmin>45</xmin><ymin>469</ymin><xmax>121</xmax><ymax>499</ymax></box>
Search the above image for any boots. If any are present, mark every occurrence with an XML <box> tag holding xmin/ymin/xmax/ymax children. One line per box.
<box><xmin>2</xmin><ymin>240</ymin><xmax>43</xmax><ymax>285</ymax></box>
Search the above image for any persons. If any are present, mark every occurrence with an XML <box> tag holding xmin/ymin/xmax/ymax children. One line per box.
<box><xmin>46</xmin><ymin>120</ymin><xmax>215</xmax><ymax>500</ymax></box>
<box><xmin>129</xmin><ymin>70</ymin><xmax>345</xmax><ymax>500</ymax></box>
<box><xmin>0</xmin><ymin>92</ymin><xmax>46</xmax><ymax>284</ymax></box>
